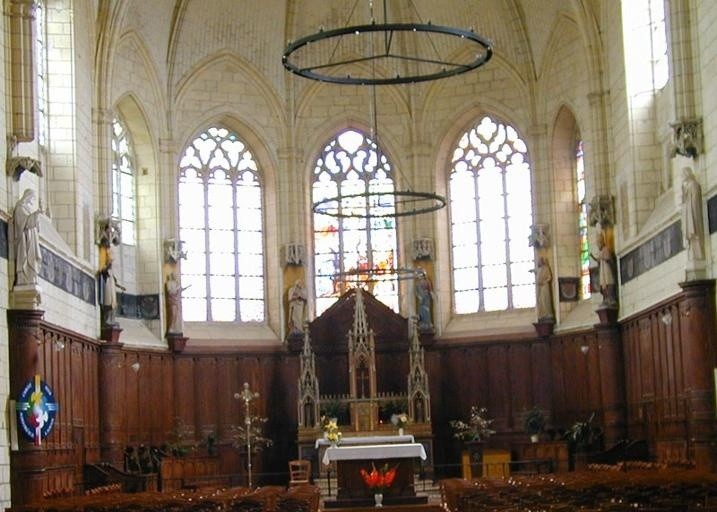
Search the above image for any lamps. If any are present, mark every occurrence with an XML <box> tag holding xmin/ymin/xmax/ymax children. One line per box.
<box><xmin>580</xmin><ymin>335</ymin><xmax>590</xmax><ymax>355</ymax></box>
<box><xmin>37</xmin><ymin>336</ymin><xmax>65</xmax><ymax>352</ymax></box>
<box><xmin>130</xmin><ymin>361</ymin><xmax>139</xmax><ymax>371</ymax></box>
<box><xmin>661</xmin><ymin>307</ymin><xmax>671</xmax><ymax>325</ymax></box>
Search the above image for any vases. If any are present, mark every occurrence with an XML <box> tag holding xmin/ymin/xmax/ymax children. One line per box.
<box><xmin>330</xmin><ymin>440</ymin><xmax>337</xmax><ymax>447</ymax></box>
<box><xmin>399</xmin><ymin>428</ymin><xmax>403</xmax><ymax>435</ymax></box>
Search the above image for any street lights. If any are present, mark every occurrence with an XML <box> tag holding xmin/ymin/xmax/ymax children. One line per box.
<box><xmin>234</xmin><ymin>382</ymin><xmax>260</xmax><ymax>491</ymax></box>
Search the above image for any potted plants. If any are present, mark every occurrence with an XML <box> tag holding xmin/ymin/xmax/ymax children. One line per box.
<box><xmin>561</xmin><ymin>414</ymin><xmax>600</xmax><ymax>471</ymax></box>
<box><xmin>449</xmin><ymin>405</ymin><xmax>500</xmax><ymax>477</ymax></box>
<box><xmin>524</xmin><ymin>406</ymin><xmax>544</xmax><ymax>442</ymax></box>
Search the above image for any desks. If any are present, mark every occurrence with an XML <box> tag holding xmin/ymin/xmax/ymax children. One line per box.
<box><xmin>322</xmin><ymin>436</ymin><xmax>426</xmax><ymax>495</ymax></box>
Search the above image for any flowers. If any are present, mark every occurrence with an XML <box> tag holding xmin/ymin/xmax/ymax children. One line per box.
<box><xmin>392</xmin><ymin>414</ymin><xmax>408</xmax><ymax>427</ymax></box>
<box><xmin>325</xmin><ymin>421</ymin><xmax>338</xmax><ymax>441</ymax></box>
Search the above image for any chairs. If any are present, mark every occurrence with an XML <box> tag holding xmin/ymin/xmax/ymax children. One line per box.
<box><xmin>288</xmin><ymin>460</ymin><xmax>312</xmax><ymax>487</ymax></box>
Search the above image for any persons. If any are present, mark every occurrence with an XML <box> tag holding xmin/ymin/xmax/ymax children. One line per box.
<box><xmin>12</xmin><ymin>188</ymin><xmax>44</xmax><ymax>284</ymax></box>
<box><xmin>587</xmin><ymin>234</ymin><xmax>617</xmax><ymax>306</ymax></box>
<box><xmin>674</xmin><ymin>167</ymin><xmax>705</xmax><ymax>262</ymax></box>
<box><xmin>527</xmin><ymin>256</ymin><xmax>554</xmax><ymax>320</ymax></box>
<box><xmin>165</xmin><ymin>273</ymin><xmax>192</xmax><ymax>334</ymax></box>
<box><xmin>413</xmin><ymin>272</ymin><xmax>435</xmax><ymax>328</ymax></box>
<box><xmin>101</xmin><ymin>259</ymin><xmax>127</xmax><ymax>328</ymax></box>
<box><xmin>285</xmin><ymin>278</ymin><xmax>307</xmax><ymax>337</ymax></box>
